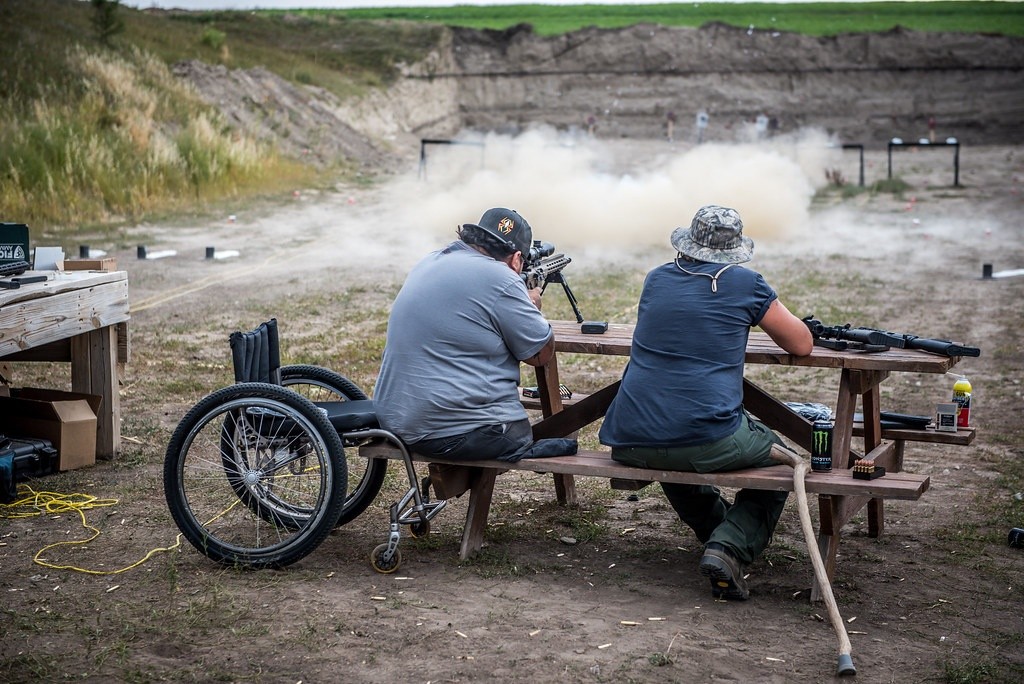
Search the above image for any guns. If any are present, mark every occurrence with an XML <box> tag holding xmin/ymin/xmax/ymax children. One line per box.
<box><xmin>518</xmin><ymin>240</ymin><xmax>584</xmax><ymax>324</ymax></box>
<box><xmin>802</xmin><ymin>315</ymin><xmax>982</xmax><ymax>357</ymax></box>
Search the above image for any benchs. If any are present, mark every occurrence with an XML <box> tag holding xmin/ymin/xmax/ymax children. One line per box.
<box><xmin>359</xmin><ymin>319</ymin><xmax>975</xmax><ymax>601</ymax></box>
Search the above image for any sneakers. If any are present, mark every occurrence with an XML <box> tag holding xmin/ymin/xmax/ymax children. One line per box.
<box><xmin>697</xmin><ymin>545</ymin><xmax>752</xmax><ymax>602</ymax></box>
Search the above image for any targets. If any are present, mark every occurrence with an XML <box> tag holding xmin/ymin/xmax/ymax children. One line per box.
<box><xmin>697</xmin><ymin>112</ymin><xmax>708</xmax><ymax>128</ymax></box>
<box><xmin>756</xmin><ymin>116</ymin><xmax>768</xmax><ymax>133</ymax></box>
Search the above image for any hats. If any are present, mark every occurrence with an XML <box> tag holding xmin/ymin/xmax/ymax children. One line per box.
<box><xmin>669</xmin><ymin>205</ymin><xmax>755</xmax><ymax>265</ymax></box>
<box><xmin>461</xmin><ymin>207</ymin><xmax>532</xmax><ymax>266</ymax></box>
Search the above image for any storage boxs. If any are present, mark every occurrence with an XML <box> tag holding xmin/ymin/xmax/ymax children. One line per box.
<box><xmin>0</xmin><ymin>385</ymin><xmax>103</xmax><ymax>471</ymax></box>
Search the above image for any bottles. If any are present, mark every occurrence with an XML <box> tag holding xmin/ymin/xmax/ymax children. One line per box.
<box><xmin>953</xmin><ymin>374</ymin><xmax>973</xmax><ymax>427</ymax></box>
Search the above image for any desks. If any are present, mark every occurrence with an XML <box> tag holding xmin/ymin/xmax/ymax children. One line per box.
<box><xmin>418</xmin><ymin>140</ymin><xmax>487</xmax><ymax>180</ymax></box>
<box><xmin>888</xmin><ymin>136</ymin><xmax>958</xmax><ymax>186</ymax></box>
<box><xmin>795</xmin><ymin>147</ymin><xmax>865</xmax><ymax>188</ymax></box>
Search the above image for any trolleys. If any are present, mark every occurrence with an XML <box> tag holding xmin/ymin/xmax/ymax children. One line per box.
<box><xmin>161</xmin><ymin>317</ymin><xmax>449</xmax><ymax>572</ymax></box>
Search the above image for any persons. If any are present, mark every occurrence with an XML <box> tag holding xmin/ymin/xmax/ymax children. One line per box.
<box><xmin>597</xmin><ymin>204</ymin><xmax>812</xmax><ymax>600</ymax></box>
<box><xmin>372</xmin><ymin>206</ymin><xmax>580</xmax><ymax>460</ymax></box>
